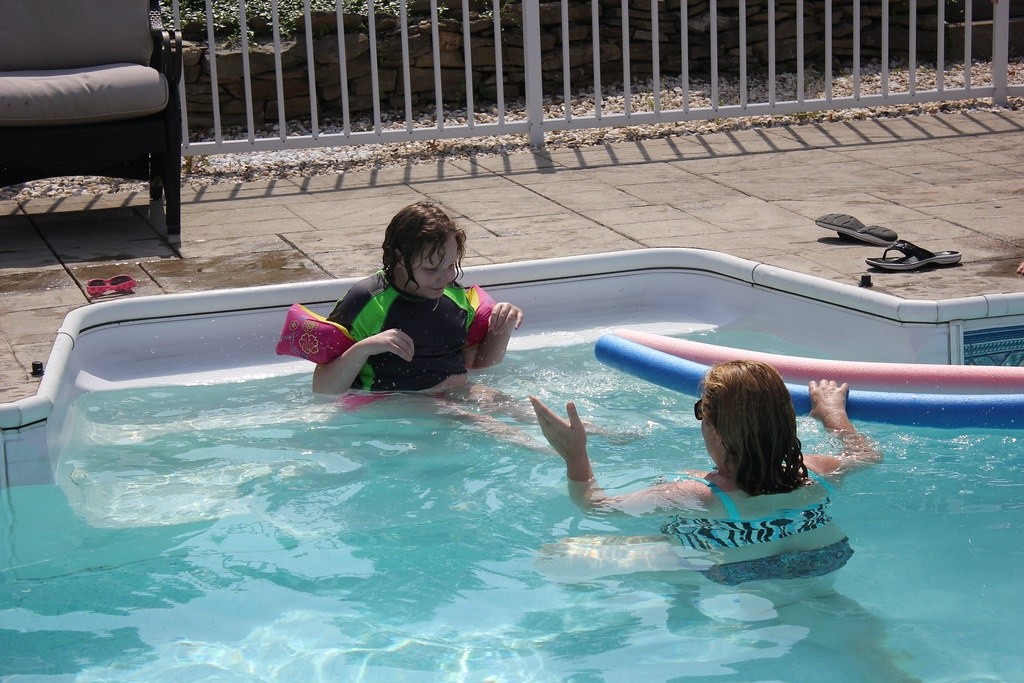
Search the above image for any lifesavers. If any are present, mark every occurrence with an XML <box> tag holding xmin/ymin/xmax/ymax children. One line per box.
<box><xmin>274</xmin><ymin>287</ymin><xmax>499</xmax><ymax>410</ymax></box>
<box><xmin>613</xmin><ymin>325</ymin><xmax>1024</xmax><ymax>394</ymax></box>
<box><xmin>595</xmin><ymin>333</ymin><xmax>1024</xmax><ymax>430</ymax></box>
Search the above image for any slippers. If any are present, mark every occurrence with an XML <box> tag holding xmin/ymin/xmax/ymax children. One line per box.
<box><xmin>86</xmin><ymin>273</ymin><xmax>137</xmax><ymax>297</ymax></box>
<box><xmin>814</xmin><ymin>213</ymin><xmax>898</xmax><ymax>246</ymax></box>
<box><xmin>866</xmin><ymin>239</ymin><xmax>962</xmax><ymax>272</ymax></box>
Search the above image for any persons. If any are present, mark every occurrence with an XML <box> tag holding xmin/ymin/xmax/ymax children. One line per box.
<box><xmin>527</xmin><ymin>361</ymin><xmax>882</xmax><ymax>591</ymax></box>
<box><xmin>311</xmin><ymin>199</ymin><xmax>645</xmax><ymax>459</ymax></box>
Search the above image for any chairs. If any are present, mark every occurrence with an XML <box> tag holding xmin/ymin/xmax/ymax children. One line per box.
<box><xmin>0</xmin><ymin>0</ymin><xmax>183</xmax><ymax>235</ymax></box>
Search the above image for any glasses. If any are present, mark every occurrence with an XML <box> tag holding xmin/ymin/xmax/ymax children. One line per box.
<box><xmin>694</xmin><ymin>399</ymin><xmax>711</xmax><ymax>431</ymax></box>
